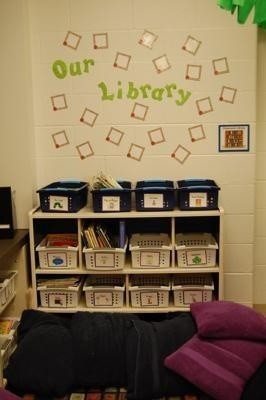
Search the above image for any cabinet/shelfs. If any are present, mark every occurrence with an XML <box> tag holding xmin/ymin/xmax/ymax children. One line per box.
<box><xmin>28</xmin><ymin>204</ymin><xmax>224</xmax><ymax>314</ymax></box>
<box><xmin>0</xmin><ymin>229</ymin><xmax>32</xmax><ymax>399</ymax></box>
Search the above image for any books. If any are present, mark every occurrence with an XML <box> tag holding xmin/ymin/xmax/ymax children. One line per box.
<box><xmin>96</xmin><ymin>168</ymin><xmax>123</xmax><ymax>189</ymax></box>
<box><xmin>81</xmin><ymin>224</ymin><xmax>115</xmax><ymax>249</ymax></box>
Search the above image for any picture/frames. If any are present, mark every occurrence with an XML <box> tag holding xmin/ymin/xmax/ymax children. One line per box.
<box><xmin>219</xmin><ymin>124</ymin><xmax>250</xmax><ymax>152</ymax></box>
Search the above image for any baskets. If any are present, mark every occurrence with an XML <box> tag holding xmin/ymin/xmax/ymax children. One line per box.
<box><xmin>36</xmin><ymin>234</ymin><xmax>78</xmax><ymax>269</ymax></box>
<box><xmin>176</xmin><ymin>179</ymin><xmax>220</xmax><ymax>210</ymax></box>
<box><xmin>175</xmin><ymin>233</ymin><xmax>219</xmax><ymax>267</ymax></box>
<box><xmin>36</xmin><ymin>181</ymin><xmax>87</xmax><ymax>212</ymax></box>
<box><xmin>90</xmin><ymin>180</ymin><xmax>131</xmax><ymax>212</ymax></box>
<box><xmin>82</xmin><ymin>236</ymin><xmax>128</xmax><ymax>270</ymax></box>
<box><xmin>129</xmin><ymin>233</ymin><xmax>172</xmax><ymax>268</ymax></box>
<box><xmin>172</xmin><ymin>274</ymin><xmax>214</xmax><ymax>306</ymax></box>
<box><xmin>37</xmin><ymin>276</ymin><xmax>83</xmax><ymax>307</ymax></box>
<box><xmin>128</xmin><ymin>274</ymin><xmax>171</xmax><ymax>308</ymax></box>
<box><xmin>82</xmin><ymin>274</ymin><xmax>125</xmax><ymax>308</ymax></box>
<box><xmin>0</xmin><ymin>317</ymin><xmax>21</xmax><ymax>389</ymax></box>
<box><xmin>0</xmin><ymin>270</ymin><xmax>17</xmax><ymax>314</ymax></box>
<box><xmin>132</xmin><ymin>180</ymin><xmax>175</xmax><ymax>210</ymax></box>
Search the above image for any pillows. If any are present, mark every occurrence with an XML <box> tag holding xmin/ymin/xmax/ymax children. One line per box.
<box><xmin>0</xmin><ymin>300</ymin><xmax>266</xmax><ymax>400</ymax></box>
